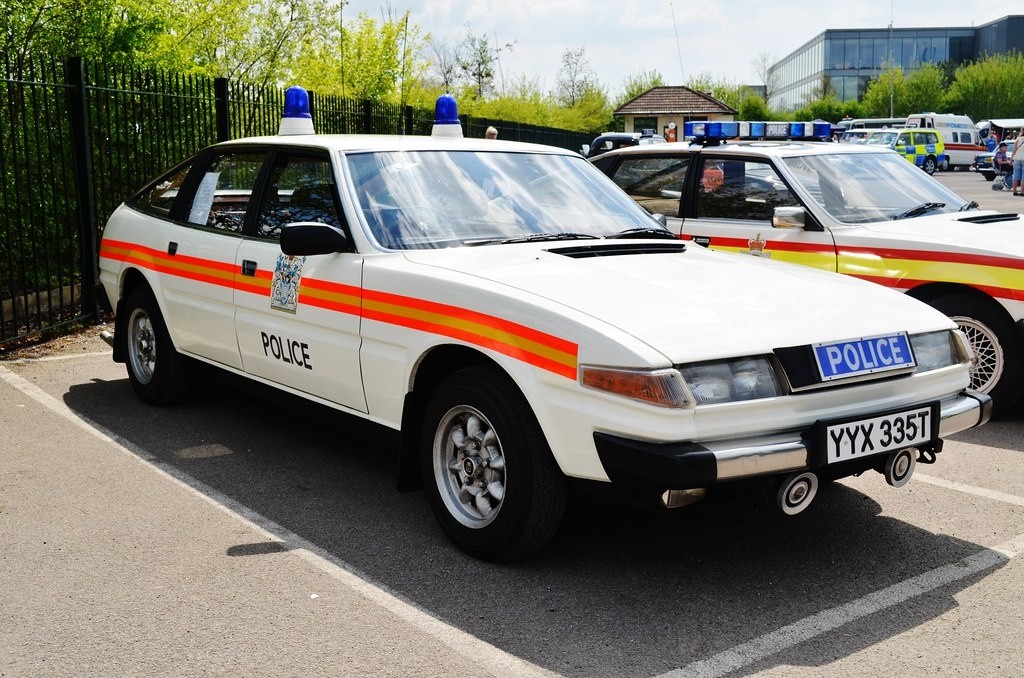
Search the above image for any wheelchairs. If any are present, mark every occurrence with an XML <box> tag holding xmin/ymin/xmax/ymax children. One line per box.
<box><xmin>991</xmin><ymin>157</ymin><xmax>1014</xmax><ymax>191</ymax></box>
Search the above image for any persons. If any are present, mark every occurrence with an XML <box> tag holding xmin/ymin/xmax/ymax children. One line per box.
<box><xmin>986</xmin><ymin>128</ymin><xmax>1017</xmax><ymax>152</ymax></box>
<box><xmin>880</xmin><ymin>126</ymin><xmax>892</xmax><ymax>144</ymax></box>
<box><xmin>994</xmin><ymin>143</ymin><xmax>1014</xmax><ymax>190</ymax></box>
<box><xmin>485</xmin><ymin>126</ymin><xmax>497</xmax><ymax>139</ymax></box>
<box><xmin>1009</xmin><ymin>127</ymin><xmax>1024</xmax><ymax>197</ymax></box>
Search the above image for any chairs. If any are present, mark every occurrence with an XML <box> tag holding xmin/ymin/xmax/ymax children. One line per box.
<box><xmin>764</xmin><ymin>190</ymin><xmax>804</xmax><ymax>214</ymax></box>
<box><xmin>289</xmin><ymin>185</ymin><xmax>341</xmax><ymax>232</ymax></box>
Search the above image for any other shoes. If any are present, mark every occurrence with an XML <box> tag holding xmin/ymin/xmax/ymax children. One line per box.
<box><xmin>1013</xmin><ymin>192</ymin><xmax>1024</xmax><ymax>196</ymax></box>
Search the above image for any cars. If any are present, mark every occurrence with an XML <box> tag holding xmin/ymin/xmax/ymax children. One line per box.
<box><xmin>491</xmin><ymin>119</ymin><xmax>1024</xmax><ymax>424</ymax></box>
<box><xmin>97</xmin><ymin>86</ymin><xmax>995</xmax><ymax>564</ymax></box>
<box><xmin>971</xmin><ymin>137</ymin><xmax>1017</xmax><ymax>181</ymax></box>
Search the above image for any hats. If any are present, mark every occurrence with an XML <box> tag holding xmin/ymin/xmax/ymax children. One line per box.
<box><xmin>999</xmin><ymin>143</ymin><xmax>1009</xmax><ymax>148</ymax></box>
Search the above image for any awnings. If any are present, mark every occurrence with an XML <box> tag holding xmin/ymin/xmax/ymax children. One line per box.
<box><xmin>990</xmin><ymin>121</ymin><xmax>1024</xmax><ymax>129</ymax></box>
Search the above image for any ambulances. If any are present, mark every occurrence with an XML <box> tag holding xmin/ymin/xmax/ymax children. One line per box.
<box><xmin>862</xmin><ymin>123</ymin><xmax>946</xmax><ymax>177</ymax></box>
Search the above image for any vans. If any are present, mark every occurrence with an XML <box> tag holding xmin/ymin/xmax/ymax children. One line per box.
<box><xmin>586</xmin><ymin>128</ymin><xmax>669</xmax><ymax>160</ymax></box>
<box><xmin>834</xmin><ymin>109</ymin><xmax>991</xmax><ymax>172</ymax></box>
